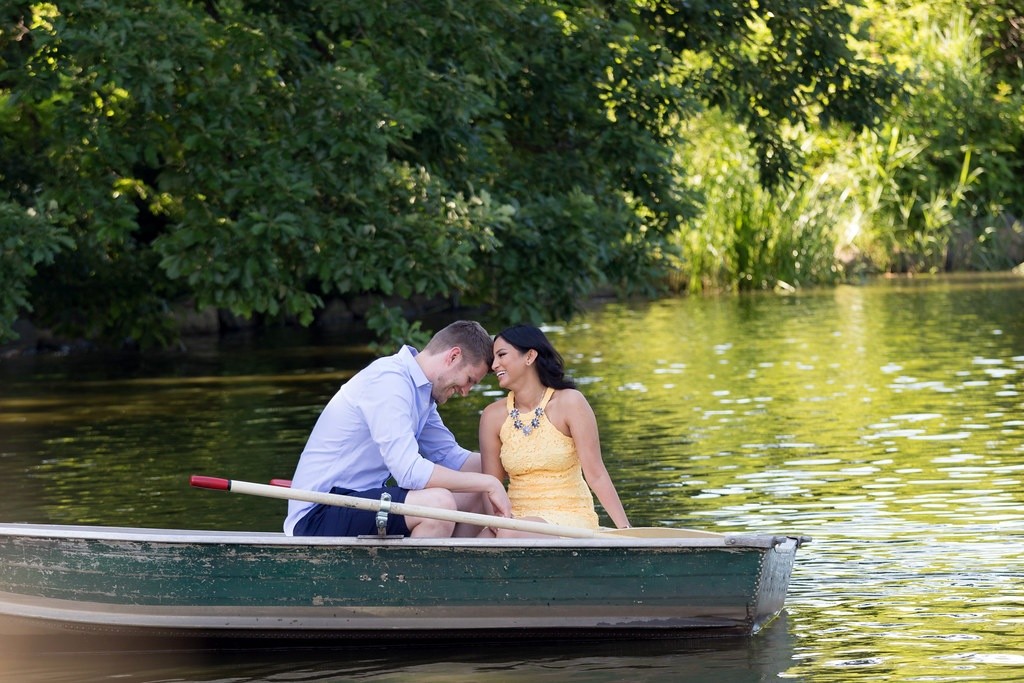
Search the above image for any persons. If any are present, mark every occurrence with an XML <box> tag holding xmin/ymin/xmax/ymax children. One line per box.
<box><xmin>282</xmin><ymin>320</ymin><xmax>511</xmax><ymax>537</ymax></box>
<box><xmin>475</xmin><ymin>326</ymin><xmax>633</xmax><ymax>539</ymax></box>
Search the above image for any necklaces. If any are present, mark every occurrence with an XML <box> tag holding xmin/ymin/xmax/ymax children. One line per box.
<box><xmin>510</xmin><ymin>386</ymin><xmax>546</xmax><ymax>436</ymax></box>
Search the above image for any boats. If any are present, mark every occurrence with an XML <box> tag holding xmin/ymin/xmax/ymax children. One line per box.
<box><xmin>0</xmin><ymin>521</ymin><xmax>813</xmax><ymax>632</ymax></box>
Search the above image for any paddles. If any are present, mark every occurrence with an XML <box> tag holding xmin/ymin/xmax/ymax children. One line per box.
<box><xmin>191</xmin><ymin>475</ymin><xmax>729</xmax><ymax>538</ymax></box>
